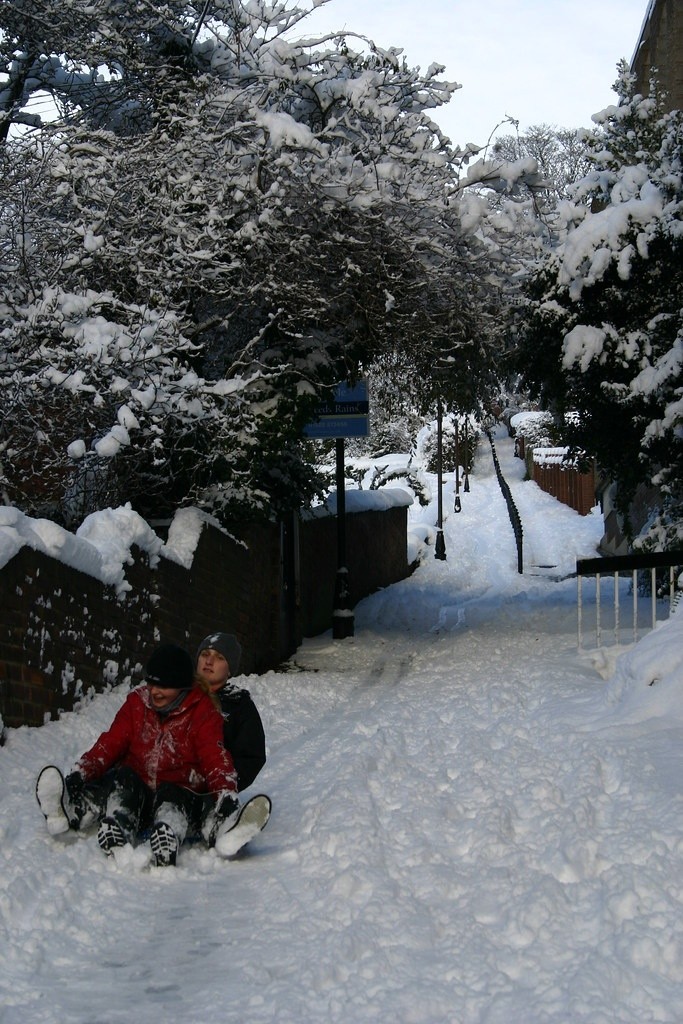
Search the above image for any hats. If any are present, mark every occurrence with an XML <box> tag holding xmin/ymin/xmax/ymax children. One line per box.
<box><xmin>198</xmin><ymin>631</ymin><xmax>242</xmax><ymax>679</ymax></box>
<box><xmin>145</xmin><ymin>647</ymin><xmax>196</xmax><ymax>689</ymax></box>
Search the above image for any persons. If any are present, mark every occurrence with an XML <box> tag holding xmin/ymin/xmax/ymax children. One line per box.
<box><xmin>36</xmin><ymin>632</ymin><xmax>273</xmax><ymax>858</ymax></box>
<box><xmin>63</xmin><ymin>642</ymin><xmax>240</xmax><ymax>867</ymax></box>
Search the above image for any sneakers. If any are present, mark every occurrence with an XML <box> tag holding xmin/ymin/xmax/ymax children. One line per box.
<box><xmin>35</xmin><ymin>765</ymin><xmax>70</xmax><ymax>836</ymax></box>
<box><xmin>97</xmin><ymin>816</ymin><xmax>132</xmax><ymax>863</ymax></box>
<box><xmin>214</xmin><ymin>794</ymin><xmax>272</xmax><ymax>856</ymax></box>
<box><xmin>150</xmin><ymin>821</ymin><xmax>178</xmax><ymax>867</ymax></box>
